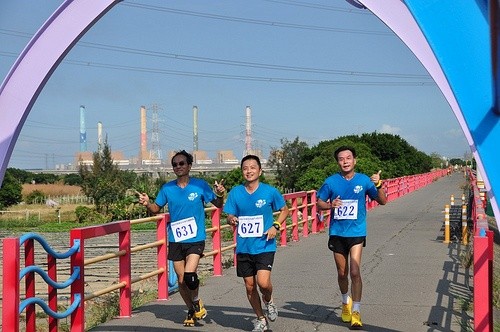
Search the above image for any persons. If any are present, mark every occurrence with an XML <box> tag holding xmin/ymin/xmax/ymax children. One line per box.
<box><xmin>317</xmin><ymin>146</ymin><xmax>387</xmax><ymax>327</ymax></box>
<box><xmin>135</xmin><ymin>151</ymin><xmax>225</xmax><ymax>327</ymax></box>
<box><xmin>222</xmin><ymin>155</ymin><xmax>289</xmax><ymax>332</ymax></box>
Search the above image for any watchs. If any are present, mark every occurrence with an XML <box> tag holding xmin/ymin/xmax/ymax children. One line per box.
<box><xmin>272</xmin><ymin>224</ymin><xmax>280</xmax><ymax>230</ymax></box>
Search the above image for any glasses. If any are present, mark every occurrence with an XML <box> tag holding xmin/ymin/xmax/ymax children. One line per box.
<box><xmin>173</xmin><ymin>161</ymin><xmax>187</xmax><ymax>167</ymax></box>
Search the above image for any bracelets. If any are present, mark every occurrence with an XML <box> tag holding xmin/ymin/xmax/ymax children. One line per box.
<box><xmin>274</xmin><ymin>222</ymin><xmax>281</xmax><ymax>227</ymax></box>
<box><xmin>216</xmin><ymin>194</ymin><xmax>224</xmax><ymax>197</ymax></box>
<box><xmin>376</xmin><ymin>180</ymin><xmax>382</xmax><ymax>189</ymax></box>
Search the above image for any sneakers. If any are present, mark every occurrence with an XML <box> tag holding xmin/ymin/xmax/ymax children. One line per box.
<box><xmin>341</xmin><ymin>296</ymin><xmax>353</xmax><ymax>323</ymax></box>
<box><xmin>262</xmin><ymin>294</ymin><xmax>278</xmax><ymax>322</ymax></box>
<box><xmin>252</xmin><ymin>317</ymin><xmax>268</xmax><ymax>332</ymax></box>
<box><xmin>191</xmin><ymin>298</ymin><xmax>207</xmax><ymax>319</ymax></box>
<box><xmin>351</xmin><ymin>311</ymin><xmax>362</xmax><ymax>329</ymax></box>
<box><xmin>183</xmin><ymin>309</ymin><xmax>198</xmax><ymax>326</ymax></box>
